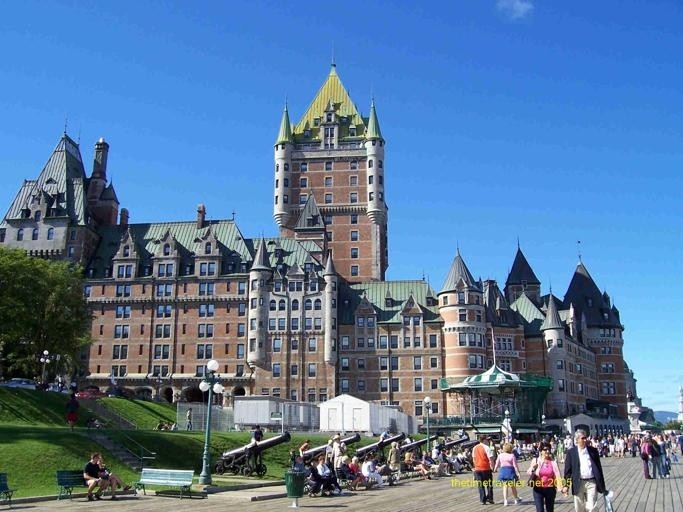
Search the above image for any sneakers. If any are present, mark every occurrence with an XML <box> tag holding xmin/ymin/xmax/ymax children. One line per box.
<box><xmin>504</xmin><ymin>500</ymin><xmax>508</xmax><ymax>505</ymax></box>
<box><xmin>515</xmin><ymin>497</ymin><xmax>522</xmax><ymax>504</ymax></box>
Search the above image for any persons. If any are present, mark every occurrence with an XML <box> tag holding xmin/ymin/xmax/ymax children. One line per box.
<box><xmin>35</xmin><ymin>376</ymin><xmax>80</xmax><ymax>401</ymax></box>
<box><xmin>66</xmin><ymin>394</ymin><xmax>80</xmax><ymax>425</ymax></box>
<box><xmin>83</xmin><ymin>454</ymin><xmax>132</xmax><ymax>500</ymax></box>
<box><xmin>111</xmin><ymin>385</ymin><xmax>152</xmax><ymax>400</ymax></box>
<box><xmin>88</xmin><ymin>418</ymin><xmax>96</xmax><ymax>437</ymax></box>
<box><xmin>254</xmin><ymin>425</ymin><xmax>263</xmax><ymax>465</ymax></box>
<box><xmin>157</xmin><ymin>420</ymin><xmax>176</xmax><ymax>431</ymax></box>
<box><xmin>186</xmin><ymin>408</ymin><xmax>192</xmax><ymax>430</ymax></box>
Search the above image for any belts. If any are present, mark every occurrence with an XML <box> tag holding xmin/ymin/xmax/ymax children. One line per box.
<box><xmin>581</xmin><ymin>478</ymin><xmax>594</xmax><ymax>481</ymax></box>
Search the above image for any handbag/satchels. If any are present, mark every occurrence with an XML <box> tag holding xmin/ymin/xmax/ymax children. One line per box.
<box><xmin>527</xmin><ymin>457</ymin><xmax>537</xmax><ymax>487</ymax></box>
<box><xmin>604</xmin><ymin>491</ymin><xmax>613</xmax><ymax>512</ymax></box>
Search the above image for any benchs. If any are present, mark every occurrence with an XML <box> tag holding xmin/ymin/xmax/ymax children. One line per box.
<box><xmin>133</xmin><ymin>469</ymin><xmax>194</xmax><ymax>499</ymax></box>
<box><xmin>304</xmin><ymin>460</ymin><xmax>419</xmax><ymax>496</ymax></box>
<box><xmin>57</xmin><ymin>470</ymin><xmax>87</xmax><ymax>501</ymax></box>
<box><xmin>0</xmin><ymin>473</ymin><xmax>16</xmax><ymax>509</ymax></box>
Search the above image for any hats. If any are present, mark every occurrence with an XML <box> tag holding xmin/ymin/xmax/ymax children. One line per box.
<box><xmin>480</xmin><ymin>435</ymin><xmax>491</xmax><ymax>440</ymax></box>
<box><xmin>333</xmin><ymin>435</ymin><xmax>340</xmax><ymax>440</ymax></box>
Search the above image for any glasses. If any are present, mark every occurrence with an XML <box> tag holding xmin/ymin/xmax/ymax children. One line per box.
<box><xmin>543</xmin><ymin>449</ymin><xmax>550</xmax><ymax>451</ymax></box>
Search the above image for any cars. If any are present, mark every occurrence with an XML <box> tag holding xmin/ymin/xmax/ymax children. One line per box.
<box><xmin>1</xmin><ymin>376</ymin><xmax>153</xmax><ymax>401</ymax></box>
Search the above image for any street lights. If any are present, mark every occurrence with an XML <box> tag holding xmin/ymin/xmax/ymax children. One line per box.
<box><xmin>541</xmin><ymin>414</ymin><xmax>546</xmax><ymax>440</ymax></box>
<box><xmin>424</xmin><ymin>396</ymin><xmax>433</xmax><ymax>456</ymax></box>
<box><xmin>155</xmin><ymin>374</ymin><xmax>162</xmax><ymax>401</ymax></box>
<box><xmin>39</xmin><ymin>350</ymin><xmax>50</xmax><ymax>392</ymax></box>
<box><xmin>198</xmin><ymin>359</ymin><xmax>222</xmax><ymax>487</ymax></box>
<box><xmin>504</xmin><ymin>410</ymin><xmax>511</xmax><ymax>443</ymax></box>
<box><xmin>563</xmin><ymin>418</ymin><xmax>568</xmax><ymax>438</ymax></box>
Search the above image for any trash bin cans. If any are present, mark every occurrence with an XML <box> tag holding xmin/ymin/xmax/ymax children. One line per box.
<box><xmin>284</xmin><ymin>471</ymin><xmax>304</xmax><ymax>498</ymax></box>
